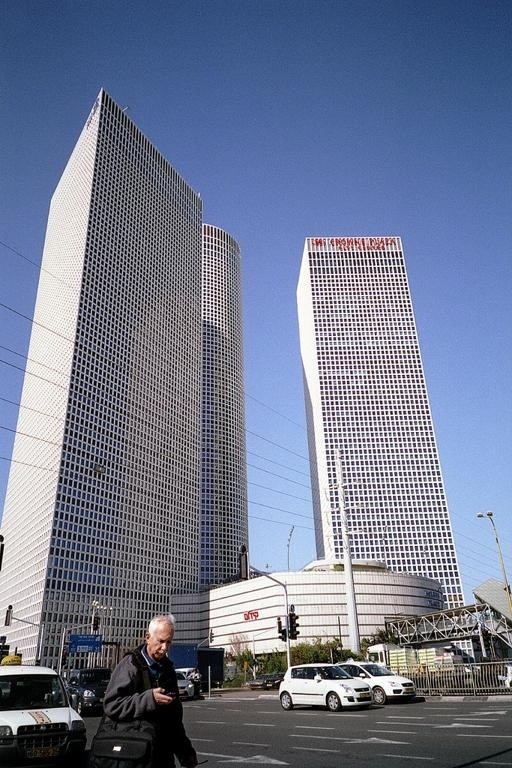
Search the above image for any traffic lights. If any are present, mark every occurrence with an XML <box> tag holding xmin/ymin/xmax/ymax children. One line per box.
<box><xmin>210</xmin><ymin>630</ymin><xmax>214</xmax><ymax>643</ymax></box>
<box><xmin>279</xmin><ymin>629</ymin><xmax>287</xmax><ymax>641</ymax></box>
<box><xmin>289</xmin><ymin>612</ymin><xmax>299</xmax><ymax>639</ymax></box>
<box><xmin>93</xmin><ymin>616</ymin><xmax>99</xmax><ymax>631</ymax></box>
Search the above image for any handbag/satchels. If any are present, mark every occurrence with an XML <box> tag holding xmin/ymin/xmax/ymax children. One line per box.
<box><xmin>88</xmin><ymin>716</ymin><xmax>154</xmax><ymax>768</ymax></box>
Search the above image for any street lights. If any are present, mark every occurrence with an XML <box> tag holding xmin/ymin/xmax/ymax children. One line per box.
<box><xmin>475</xmin><ymin>512</ymin><xmax>511</xmax><ymax>612</ymax></box>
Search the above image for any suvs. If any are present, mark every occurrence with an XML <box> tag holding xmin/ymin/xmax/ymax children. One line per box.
<box><xmin>0</xmin><ymin>665</ymin><xmax>87</xmax><ymax>760</ymax></box>
<box><xmin>65</xmin><ymin>668</ymin><xmax>111</xmax><ymax>715</ymax></box>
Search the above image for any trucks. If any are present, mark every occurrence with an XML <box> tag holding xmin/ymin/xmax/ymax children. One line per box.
<box><xmin>366</xmin><ymin>643</ymin><xmax>475</xmax><ymax>688</ymax></box>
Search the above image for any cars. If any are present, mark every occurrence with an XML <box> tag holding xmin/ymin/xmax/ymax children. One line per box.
<box><xmin>279</xmin><ymin>663</ymin><xmax>374</xmax><ymax>711</ymax></box>
<box><xmin>176</xmin><ymin>670</ymin><xmax>194</xmax><ymax>699</ymax></box>
<box><xmin>335</xmin><ymin>662</ymin><xmax>416</xmax><ymax>705</ymax></box>
<box><xmin>249</xmin><ymin>673</ymin><xmax>284</xmax><ymax>690</ymax></box>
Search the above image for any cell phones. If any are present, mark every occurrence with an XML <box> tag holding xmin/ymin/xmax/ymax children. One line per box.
<box><xmin>165</xmin><ymin>693</ymin><xmax>177</xmax><ymax>697</ymax></box>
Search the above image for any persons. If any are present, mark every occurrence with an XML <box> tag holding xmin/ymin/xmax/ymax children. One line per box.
<box><xmin>88</xmin><ymin>613</ymin><xmax>209</xmax><ymax>767</ymax></box>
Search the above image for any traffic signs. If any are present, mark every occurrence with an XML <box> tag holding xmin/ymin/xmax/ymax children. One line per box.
<box><xmin>69</xmin><ymin>634</ymin><xmax>103</xmax><ymax>653</ymax></box>
